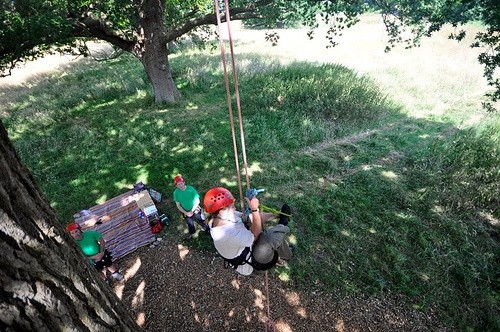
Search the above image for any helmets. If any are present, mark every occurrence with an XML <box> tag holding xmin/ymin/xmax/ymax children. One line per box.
<box><xmin>174</xmin><ymin>175</ymin><xmax>184</xmax><ymax>183</ymax></box>
<box><xmin>67</xmin><ymin>222</ymin><xmax>83</xmax><ymax>234</ymax></box>
<box><xmin>204</xmin><ymin>187</ymin><xmax>236</xmax><ymax>213</ymax></box>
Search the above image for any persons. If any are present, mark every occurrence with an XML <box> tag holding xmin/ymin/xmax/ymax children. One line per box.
<box><xmin>66</xmin><ymin>223</ymin><xmax>125</xmax><ymax>287</ymax></box>
<box><xmin>204</xmin><ymin>187</ymin><xmax>294</xmax><ymax>276</ymax></box>
<box><xmin>173</xmin><ymin>176</ymin><xmax>210</xmax><ymax>239</ymax></box>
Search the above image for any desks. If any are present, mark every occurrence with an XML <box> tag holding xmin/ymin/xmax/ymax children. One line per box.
<box><xmin>75</xmin><ymin>189</ymin><xmax>159</xmax><ymax>262</ymax></box>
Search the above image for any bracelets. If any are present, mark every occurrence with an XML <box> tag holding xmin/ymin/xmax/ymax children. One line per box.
<box><xmin>251</xmin><ymin>208</ymin><xmax>259</xmax><ymax>212</ymax></box>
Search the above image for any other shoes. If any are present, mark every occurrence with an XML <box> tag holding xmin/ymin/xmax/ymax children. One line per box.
<box><xmin>111</xmin><ymin>272</ymin><xmax>124</xmax><ymax>281</ymax></box>
<box><xmin>191</xmin><ymin>233</ymin><xmax>198</xmax><ymax>238</ymax></box>
<box><xmin>279</xmin><ymin>204</ymin><xmax>290</xmax><ymax>226</ymax></box>
